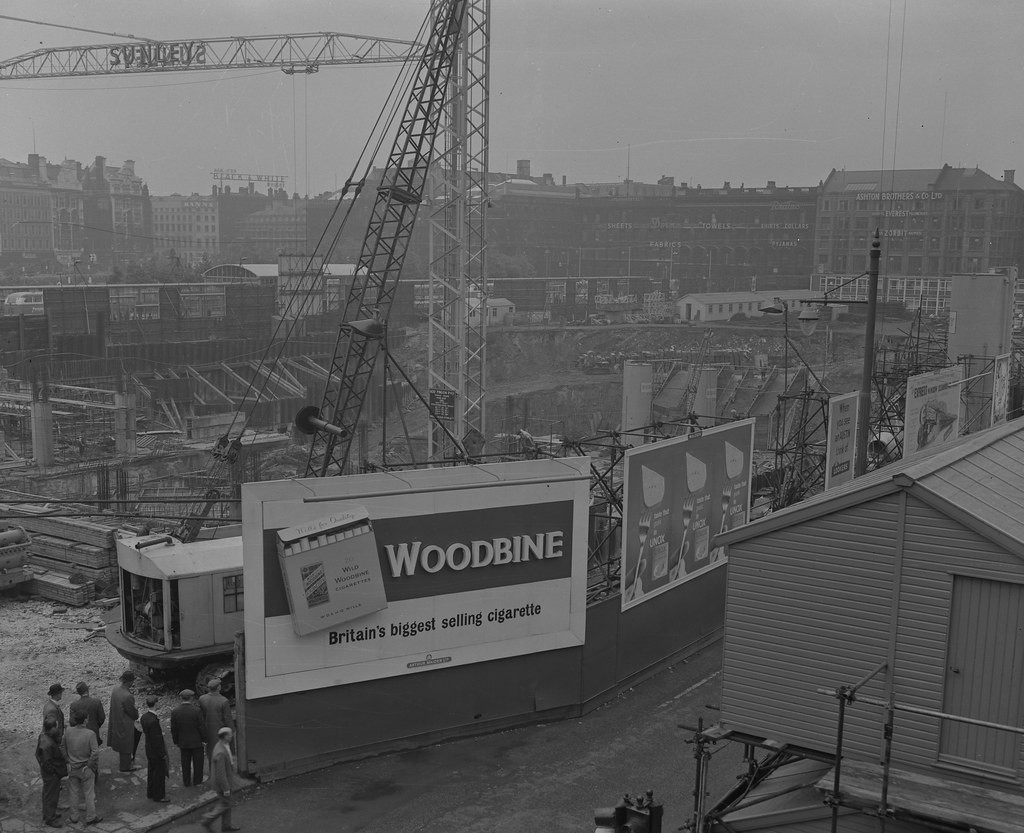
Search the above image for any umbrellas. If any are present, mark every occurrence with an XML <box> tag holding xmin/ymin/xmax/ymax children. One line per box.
<box><xmin>132</xmin><ymin>726</ymin><xmax>142</xmax><ymax>759</ymax></box>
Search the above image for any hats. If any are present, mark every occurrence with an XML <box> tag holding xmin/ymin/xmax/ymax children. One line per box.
<box><xmin>179</xmin><ymin>689</ymin><xmax>195</xmax><ymax>696</ymax></box>
<box><xmin>218</xmin><ymin>727</ymin><xmax>231</xmax><ymax>735</ymax></box>
<box><xmin>72</xmin><ymin>681</ymin><xmax>90</xmax><ymax>695</ymax></box>
<box><xmin>47</xmin><ymin>684</ymin><xmax>65</xmax><ymax>695</ymax></box>
<box><xmin>119</xmin><ymin>671</ymin><xmax>137</xmax><ymax>682</ymax></box>
<box><xmin>207</xmin><ymin>678</ymin><xmax>221</xmax><ymax>689</ymax></box>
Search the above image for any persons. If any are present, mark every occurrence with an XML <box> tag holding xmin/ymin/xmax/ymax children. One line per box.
<box><xmin>760</xmin><ymin>363</ymin><xmax>767</xmax><ymax>378</ymax></box>
<box><xmin>35</xmin><ymin>716</ymin><xmax>68</xmax><ymax>828</ymax></box>
<box><xmin>69</xmin><ymin>682</ymin><xmax>106</xmax><ymax>747</ymax></box>
<box><xmin>106</xmin><ymin>670</ymin><xmax>139</xmax><ymax>772</ymax></box>
<box><xmin>59</xmin><ymin>710</ymin><xmax>103</xmax><ymax>825</ymax></box>
<box><xmin>200</xmin><ymin>727</ymin><xmax>241</xmax><ymax>833</ymax></box>
<box><xmin>43</xmin><ymin>684</ymin><xmax>66</xmax><ymax>744</ymax></box>
<box><xmin>170</xmin><ymin>689</ymin><xmax>207</xmax><ymax>787</ymax></box>
<box><xmin>198</xmin><ymin>679</ymin><xmax>236</xmax><ymax>772</ymax></box>
<box><xmin>140</xmin><ymin>695</ymin><xmax>171</xmax><ymax>803</ymax></box>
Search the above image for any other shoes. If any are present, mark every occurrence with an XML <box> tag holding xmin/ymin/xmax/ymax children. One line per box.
<box><xmin>201</xmin><ymin>820</ymin><xmax>214</xmax><ymax>833</ymax></box>
<box><xmin>70</xmin><ymin>816</ymin><xmax>78</xmax><ymax>823</ymax></box>
<box><xmin>86</xmin><ymin>816</ymin><xmax>103</xmax><ymax>825</ymax></box>
<box><xmin>222</xmin><ymin>825</ymin><xmax>241</xmax><ymax>832</ymax></box>
<box><xmin>120</xmin><ymin>767</ymin><xmax>136</xmax><ymax>771</ymax></box>
<box><xmin>47</xmin><ymin>820</ymin><xmax>62</xmax><ymax>828</ymax></box>
<box><xmin>148</xmin><ymin>795</ymin><xmax>153</xmax><ymax>798</ymax></box>
<box><xmin>56</xmin><ymin>813</ymin><xmax>61</xmax><ymax>818</ymax></box>
<box><xmin>159</xmin><ymin>798</ymin><xmax>170</xmax><ymax>802</ymax></box>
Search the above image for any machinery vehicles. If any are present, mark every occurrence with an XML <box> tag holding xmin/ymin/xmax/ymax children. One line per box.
<box><xmin>0</xmin><ymin>0</ymin><xmax>491</xmax><ymax>707</ymax></box>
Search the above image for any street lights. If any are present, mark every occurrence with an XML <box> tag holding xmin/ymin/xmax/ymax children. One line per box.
<box><xmin>73</xmin><ymin>261</ymin><xmax>80</xmax><ymax>284</ymax></box>
<box><xmin>797</xmin><ymin>298</ymin><xmax>876</xmax><ymax>479</ymax></box>
<box><xmin>758</xmin><ymin>297</ymin><xmax>788</xmax><ymax>499</ymax></box>
